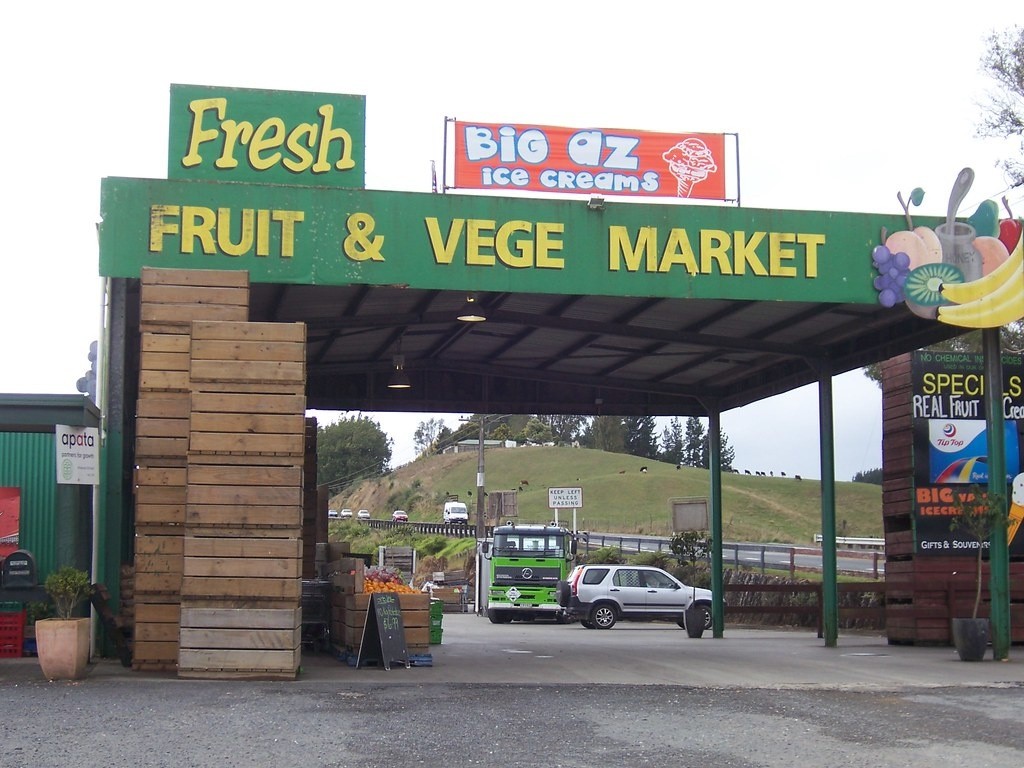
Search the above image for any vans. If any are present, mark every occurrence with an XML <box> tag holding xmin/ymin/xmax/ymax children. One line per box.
<box><xmin>443</xmin><ymin>502</ymin><xmax>468</xmax><ymax>525</ymax></box>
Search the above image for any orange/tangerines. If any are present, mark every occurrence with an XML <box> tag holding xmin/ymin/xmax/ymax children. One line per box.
<box><xmin>363</xmin><ymin>580</ymin><xmax>421</xmax><ymax>595</ymax></box>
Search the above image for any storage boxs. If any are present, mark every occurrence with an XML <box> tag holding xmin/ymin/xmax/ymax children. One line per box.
<box><xmin>0</xmin><ymin>602</ymin><xmax>35</xmax><ymax>658</ymax></box>
<box><xmin>429</xmin><ymin>601</ymin><xmax>443</xmax><ymax>643</ymax></box>
<box><xmin>322</xmin><ymin>558</ymin><xmax>430</xmax><ymax>666</ymax></box>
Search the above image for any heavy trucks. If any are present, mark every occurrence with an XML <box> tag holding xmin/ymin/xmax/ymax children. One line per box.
<box><xmin>482</xmin><ymin>520</ymin><xmax>578</xmax><ymax>624</ymax></box>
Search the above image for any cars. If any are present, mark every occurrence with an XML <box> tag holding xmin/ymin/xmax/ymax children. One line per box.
<box><xmin>392</xmin><ymin>511</ymin><xmax>409</xmax><ymax>522</ymax></box>
<box><xmin>340</xmin><ymin>509</ymin><xmax>353</xmax><ymax>518</ymax></box>
<box><xmin>328</xmin><ymin>510</ymin><xmax>338</xmax><ymax>517</ymax></box>
<box><xmin>357</xmin><ymin>510</ymin><xmax>370</xmax><ymax>519</ymax></box>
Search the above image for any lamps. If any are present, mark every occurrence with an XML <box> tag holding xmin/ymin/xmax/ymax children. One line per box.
<box><xmin>456</xmin><ymin>291</ymin><xmax>487</xmax><ymax>321</ymax></box>
<box><xmin>386</xmin><ymin>338</ymin><xmax>411</xmax><ymax>389</ymax></box>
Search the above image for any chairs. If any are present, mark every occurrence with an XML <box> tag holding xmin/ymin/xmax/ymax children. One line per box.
<box><xmin>505</xmin><ymin>541</ymin><xmax>516</xmax><ymax>548</ymax></box>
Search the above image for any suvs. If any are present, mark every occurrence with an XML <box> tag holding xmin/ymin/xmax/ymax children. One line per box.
<box><xmin>555</xmin><ymin>564</ymin><xmax>730</xmax><ymax>630</ymax></box>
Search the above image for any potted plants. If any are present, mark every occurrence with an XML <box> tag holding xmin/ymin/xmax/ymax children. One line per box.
<box><xmin>669</xmin><ymin>530</ymin><xmax>716</xmax><ymax>637</ymax></box>
<box><xmin>950</xmin><ymin>484</ymin><xmax>1018</xmax><ymax>661</ymax></box>
<box><xmin>35</xmin><ymin>565</ymin><xmax>96</xmax><ymax>681</ymax></box>
